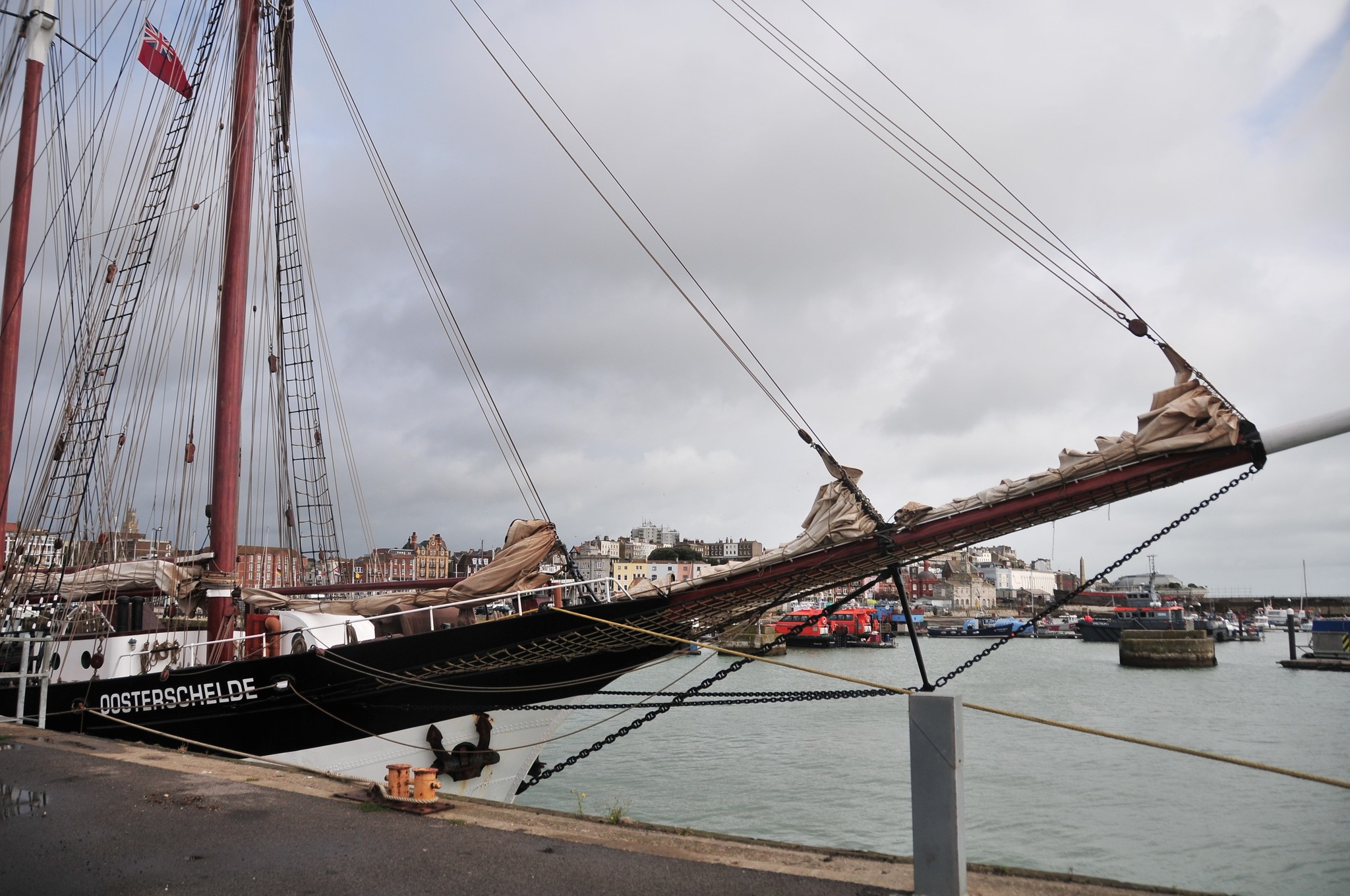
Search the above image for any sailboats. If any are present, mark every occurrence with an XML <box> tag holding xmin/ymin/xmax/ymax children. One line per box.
<box><xmin>1</xmin><ymin>0</ymin><xmax>1349</xmax><ymax>805</ymax></box>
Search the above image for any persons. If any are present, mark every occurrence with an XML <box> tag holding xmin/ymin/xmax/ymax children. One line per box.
<box><xmin>992</xmin><ymin>611</ymin><xmax>997</xmax><ymax>619</ymax></box>
<box><xmin>1058</xmin><ymin>604</ymin><xmax>1070</xmax><ymax>620</ymax></box>
<box><xmin>44</xmin><ymin>602</ymin><xmax>80</xmax><ymax>632</ymax></box>
<box><xmin>166</xmin><ymin>603</ymin><xmax>195</xmax><ymax>619</ymax></box>
<box><xmin>813</xmin><ymin>591</ymin><xmax>826</xmax><ymax>610</ymax></box>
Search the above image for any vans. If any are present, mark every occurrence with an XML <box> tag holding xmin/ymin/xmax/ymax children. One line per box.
<box><xmin>774</xmin><ymin>608</ymin><xmax>876</xmax><ymax>636</ymax></box>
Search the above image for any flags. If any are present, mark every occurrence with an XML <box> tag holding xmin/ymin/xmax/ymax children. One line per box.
<box><xmin>138</xmin><ymin>17</ymin><xmax>193</xmax><ymax>101</ymax></box>
<box><xmin>315</xmin><ymin>570</ymin><xmax>325</xmax><ymax>576</ymax></box>
<box><xmin>354</xmin><ymin>571</ymin><xmax>362</xmax><ymax>580</ymax></box>
<box><xmin>277</xmin><ymin>568</ymin><xmax>281</xmax><ymax>575</ymax></box>
<box><xmin>333</xmin><ymin>570</ymin><xmax>341</xmax><ymax>577</ymax></box>
<box><xmin>296</xmin><ymin>571</ymin><xmax>302</xmax><ymax>575</ymax></box>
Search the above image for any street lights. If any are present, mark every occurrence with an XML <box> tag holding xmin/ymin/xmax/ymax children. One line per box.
<box><xmin>149</xmin><ymin>527</ymin><xmax>163</xmax><ymax>602</ymax></box>
<box><xmin>1147</xmin><ymin>555</ymin><xmax>1151</xmax><ymax>574</ymax></box>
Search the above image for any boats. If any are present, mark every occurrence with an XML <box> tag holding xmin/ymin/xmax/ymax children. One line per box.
<box><xmin>1052</xmin><ymin>588</ymin><xmax>1128</xmax><ymax>607</ymax></box>
<box><xmin>1070</xmin><ymin>602</ymin><xmax>1245</xmax><ymax>643</ymax></box>
<box><xmin>1253</xmin><ymin>614</ymin><xmax>1274</xmax><ymax>628</ymax></box>
<box><xmin>1267</xmin><ymin>610</ymin><xmax>1300</xmax><ymax>626</ymax></box>
<box><xmin>1104</xmin><ymin>592</ymin><xmax>1161</xmax><ymax>608</ymax></box>
<box><xmin>927</xmin><ymin>601</ymin><xmax>1078</xmax><ymax>639</ymax></box>
